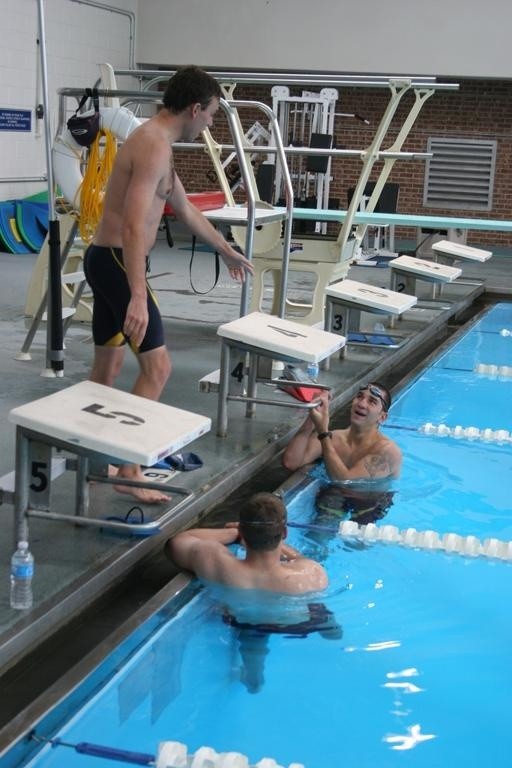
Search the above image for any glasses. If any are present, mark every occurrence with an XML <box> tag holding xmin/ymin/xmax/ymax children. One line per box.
<box><xmin>358</xmin><ymin>382</ymin><xmax>389</xmax><ymax>414</ymax></box>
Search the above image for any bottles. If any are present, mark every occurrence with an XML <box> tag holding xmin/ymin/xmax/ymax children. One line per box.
<box><xmin>307</xmin><ymin>362</ymin><xmax>319</xmax><ymax>381</ymax></box>
<box><xmin>373</xmin><ymin>320</ymin><xmax>385</xmax><ymax>333</ymax></box>
<box><xmin>10</xmin><ymin>540</ymin><xmax>34</xmax><ymax>609</ymax></box>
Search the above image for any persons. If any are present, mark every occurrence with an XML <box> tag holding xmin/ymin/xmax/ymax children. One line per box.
<box><xmin>284</xmin><ymin>382</ymin><xmax>403</xmax><ymax>561</ymax></box>
<box><xmin>164</xmin><ymin>493</ymin><xmax>343</xmax><ymax>695</ymax></box>
<box><xmin>75</xmin><ymin>66</ymin><xmax>256</xmax><ymax>505</ymax></box>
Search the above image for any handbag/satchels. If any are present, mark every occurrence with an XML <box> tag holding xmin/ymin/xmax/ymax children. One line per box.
<box><xmin>67</xmin><ymin>88</ymin><xmax>101</xmax><ymax>149</ymax></box>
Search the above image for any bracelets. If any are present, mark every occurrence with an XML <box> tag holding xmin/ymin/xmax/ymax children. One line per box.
<box><xmin>317</xmin><ymin>432</ymin><xmax>334</xmax><ymax>440</ymax></box>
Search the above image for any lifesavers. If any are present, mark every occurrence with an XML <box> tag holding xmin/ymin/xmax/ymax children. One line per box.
<box><xmin>53</xmin><ymin>107</ymin><xmax>142</xmax><ymax>211</ymax></box>
